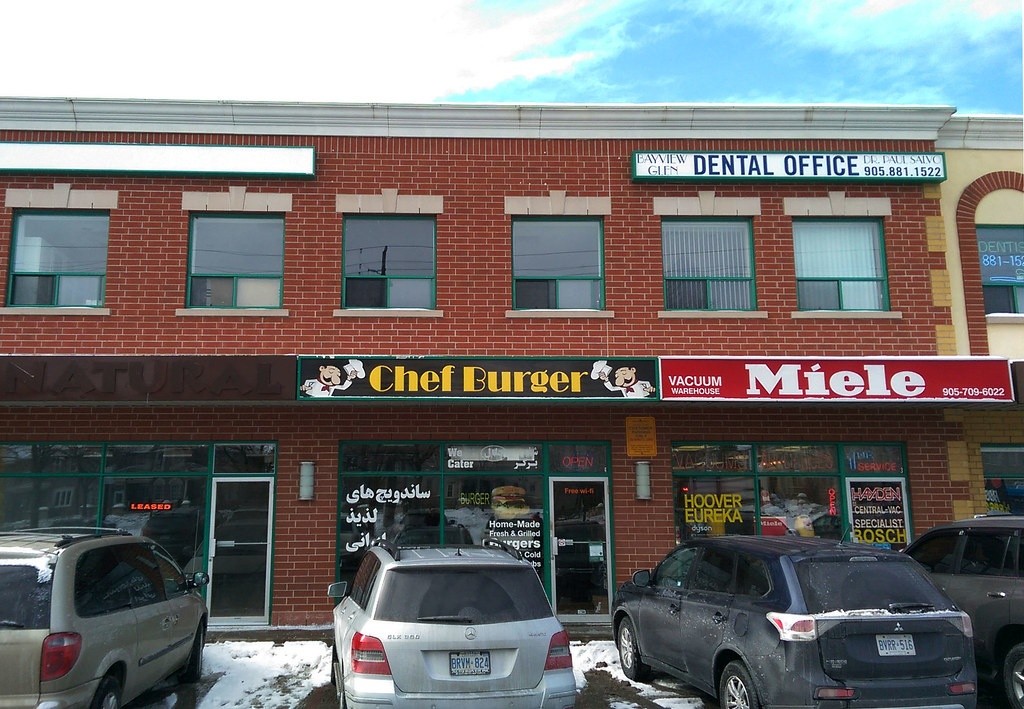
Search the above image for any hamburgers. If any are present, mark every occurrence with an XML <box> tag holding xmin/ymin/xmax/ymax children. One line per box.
<box><xmin>491</xmin><ymin>486</ymin><xmax>530</xmax><ymax>514</ymax></box>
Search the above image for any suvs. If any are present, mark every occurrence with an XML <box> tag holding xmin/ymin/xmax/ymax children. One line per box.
<box><xmin>0</xmin><ymin>525</ymin><xmax>211</xmax><ymax>709</ymax></box>
<box><xmin>611</xmin><ymin>533</ymin><xmax>978</xmax><ymax>709</ymax></box>
<box><xmin>325</xmin><ymin>536</ymin><xmax>577</xmax><ymax>709</ymax></box>
<box><xmin>339</xmin><ymin>494</ymin><xmax>608</xmax><ymax>607</ymax></box>
<box><xmin>673</xmin><ymin>498</ymin><xmax>847</xmax><ymax>547</ymax></box>
<box><xmin>0</xmin><ymin>496</ymin><xmax>268</xmax><ymax>599</ymax></box>
<box><xmin>882</xmin><ymin>512</ymin><xmax>1024</xmax><ymax>709</ymax></box>
